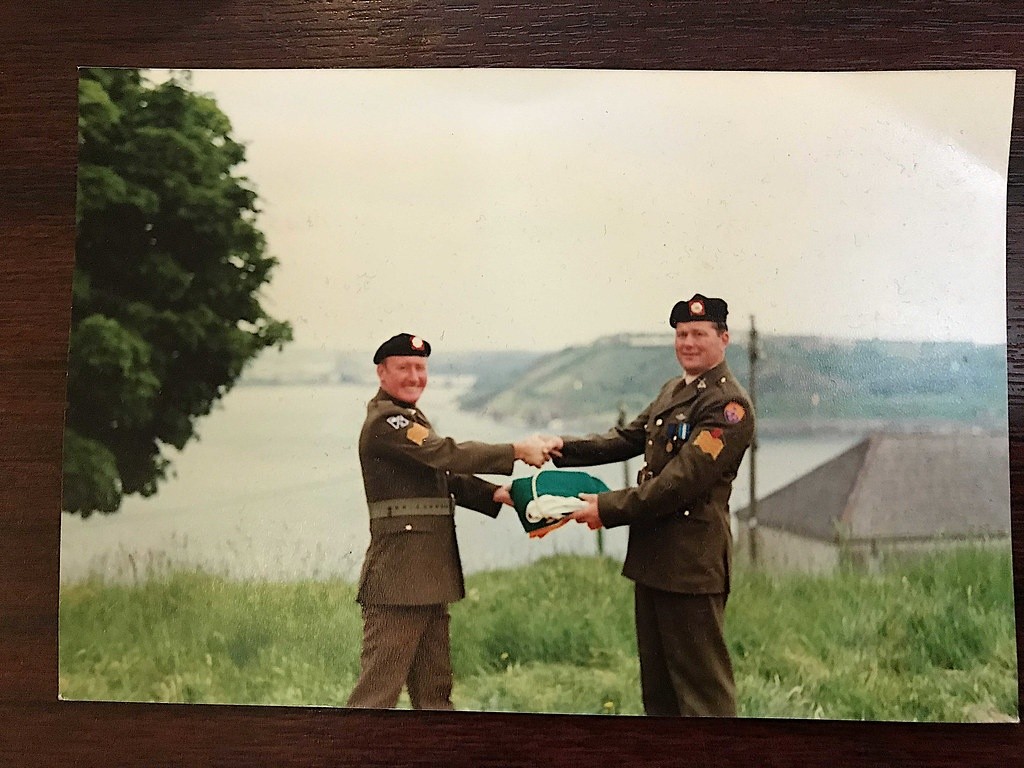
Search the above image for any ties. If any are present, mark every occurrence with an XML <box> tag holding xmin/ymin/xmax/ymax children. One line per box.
<box><xmin>672</xmin><ymin>378</ymin><xmax>687</xmax><ymax>397</ymax></box>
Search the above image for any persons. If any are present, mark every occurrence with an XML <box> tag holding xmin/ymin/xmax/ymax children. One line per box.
<box><xmin>540</xmin><ymin>294</ymin><xmax>756</xmax><ymax>717</ymax></box>
<box><xmin>347</xmin><ymin>333</ymin><xmax>562</xmax><ymax>711</ymax></box>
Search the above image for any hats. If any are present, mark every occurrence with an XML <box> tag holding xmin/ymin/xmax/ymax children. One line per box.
<box><xmin>373</xmin><ymin>332</ymin><xmax>431</xmax><ymax>364</ymax></box>
<box><xmin>669</xmin><ymin>292</ymin><xmax>729</xmax><ymax>327</ymax></box>
